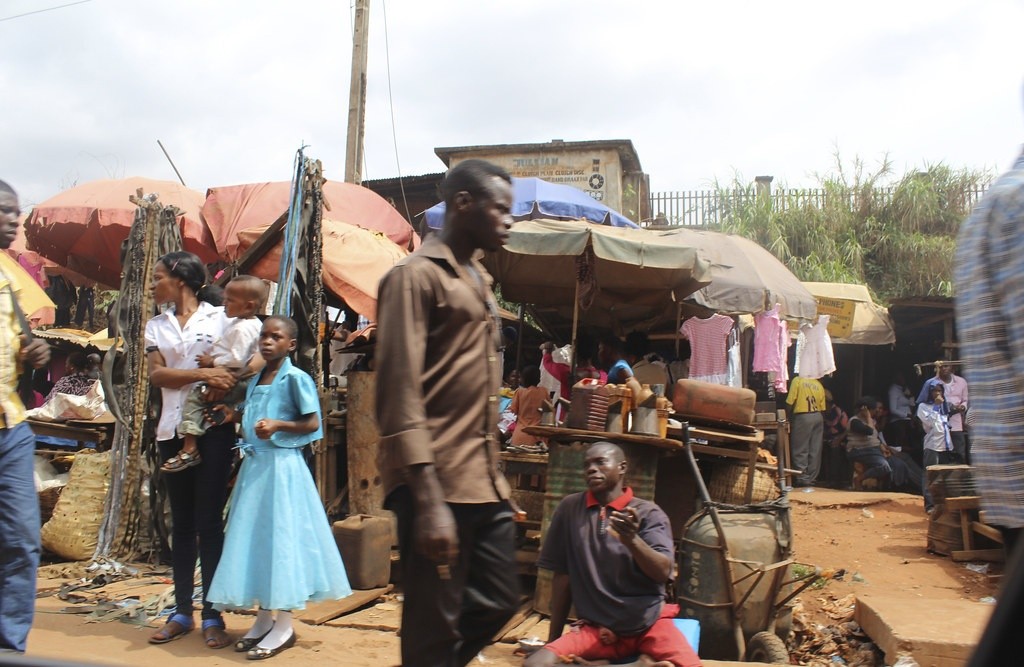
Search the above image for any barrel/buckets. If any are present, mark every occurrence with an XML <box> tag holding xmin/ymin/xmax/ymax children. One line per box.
<box><xmin>565</xmin><ymin>378</ymin><xmax>610</xmax><ymax>432</ymax></box>
<box><xmin>603</xmin><ymin>383</ymin><xmax>633</xmax><ymax>434</ymax></box>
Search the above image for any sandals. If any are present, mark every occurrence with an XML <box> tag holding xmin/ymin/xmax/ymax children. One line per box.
<box><xmin>149</xmin><ymin>612</ymin><xmax>194</xmax><ymax>643</ymax></box>
<box><xmin>160</xmin><ymin>448</ymin><xmax>202</xmax><ymax>472</ymax></box>
<box><xmin>201</xmin><ymin>616</ymin><xmax>233</xmax><ymax>648</ymax></box>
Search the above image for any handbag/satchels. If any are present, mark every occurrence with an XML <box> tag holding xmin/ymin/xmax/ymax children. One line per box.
<box><xmin>16</xmin><ymin>360</ymin><xmax>56</xmax><ymax>411</ymax></box>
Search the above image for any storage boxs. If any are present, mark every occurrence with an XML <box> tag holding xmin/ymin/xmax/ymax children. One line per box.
<box><xmin>755</xmin><ymin>400</ymin><xmax>786</xmax><ymax>422</ymax></box>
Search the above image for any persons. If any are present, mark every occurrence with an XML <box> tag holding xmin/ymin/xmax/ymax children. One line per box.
<box><xmin>16</xmin><ymin>354</ymin><xmax>102</xmax><ymax>410</ymax></box>
<box><xmin>328</xmin><ymin>321</ymin><xmax>350</xmax><ymax>359</ymax></box>
<box><xmin>375</xmin><ymin>160</ymin><xmax>522</xmax><ymax>667</ymax></box>
<box><xmin>785</xmin><ymin>376</ymin><xmax>826</xmax><ymax>488</ymax></box>
<box><xmin>951</xmin><ymin>145</ymin><xmax>1024</xmax><ymax>667</ymax></box>
<box><xmin>498</xmin><ymin>326</ymin><xmax>689</xmax><ymax>451</ymax></box>
<box><xmin>204</xmin><ymin>315</ymin><xmax>354</xmax><ymax>660</ymax></box>
<box><xmin>197</xmin><ymin>284</ymin><xmax>226</xmax><ymax>307</ymax></box>
<box><xmin>144</xmin><ymin>252</ymin><xmax>237</xmax><ymax>650</ymax></box>
<box><xmin>915</xmin><ymin>379</ymin><xmax>954</xmax><ymax>515</ymax></box>
<box><xmin>523</xmin><ymin>442</ymin><xmax>703</xmax><ymax>667</ymax></box>
<box><xmin>0</xmin><ymin>180</ymin><xmax>58</xmax><ymax>656</ymax></box>
<box><xmin>824</xmin><ymin>371</ymin><xmax>923</xmax><ymax>497</ymax></box>
<box><xmin>933</xmin><ymin>357</ymin><xmax>968</xmax><ymax>465</ymax></box>
<box><xmin>159</xmin><ymin>275</ymin><xmax>266</xmax><ymax>474</ymax></box>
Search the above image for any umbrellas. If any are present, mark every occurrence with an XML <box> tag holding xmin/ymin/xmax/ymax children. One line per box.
<box><xmin>31</xmin><ymin>327</ymin><xmax>124</xmax><ymax>353</ymax></box>
<box><xmin>413</xmin><ymin>175</ymin><xmax>818</xmax><ymax>384</ymax></box>
<box><xmin>8</xmin><ymin>177</ymin><xmax>421</xmax><ymax>326</ymax></box>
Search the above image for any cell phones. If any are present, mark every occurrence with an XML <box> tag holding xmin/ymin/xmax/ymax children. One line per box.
<box><xmin>208</xmin><ymin>403</ymin><xmax>227</xmax><ymax>425</ymax></box>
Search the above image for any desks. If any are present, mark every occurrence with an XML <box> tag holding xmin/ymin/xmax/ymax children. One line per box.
<box><xmin>25</xmin><ymin>419</ymin><xmax>116</xmax><ymax>457</ymax></box>
<box><xmin>499</xmin><ymin>450</ymin><xmax>550</xmax><ymax>577</ymax></box>
<box><xmin>521</xmin><ymin>425</ymin><xmax>767</xmax><ymax>505</ymax></box>
<box><xmin>754</xmin><ymin>420</ymin><xmax>792</xmax><ymax>487</ymax></box>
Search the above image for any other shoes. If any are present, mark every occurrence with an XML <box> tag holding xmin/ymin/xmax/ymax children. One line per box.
<box><xmin>234</xmin><ymin>626</ymin><xmax>273</xmax><ymax>652</ymax></box>
<box><xmin>248</xmin><ymin>628</ymin><xmax>296</xmax><ymax>660</ymax></box>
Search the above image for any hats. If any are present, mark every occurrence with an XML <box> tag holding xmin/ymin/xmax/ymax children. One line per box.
<box><xmin>503</xmin><ymin>326</ymin><xmax>517</xmax><ymax>342</ymax></box>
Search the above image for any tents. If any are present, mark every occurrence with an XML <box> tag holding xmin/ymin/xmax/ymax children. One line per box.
<box><xmin>739</xmin><ymin>281</ymin><xmax>896</xmax><ymax>351</ymax></box>
